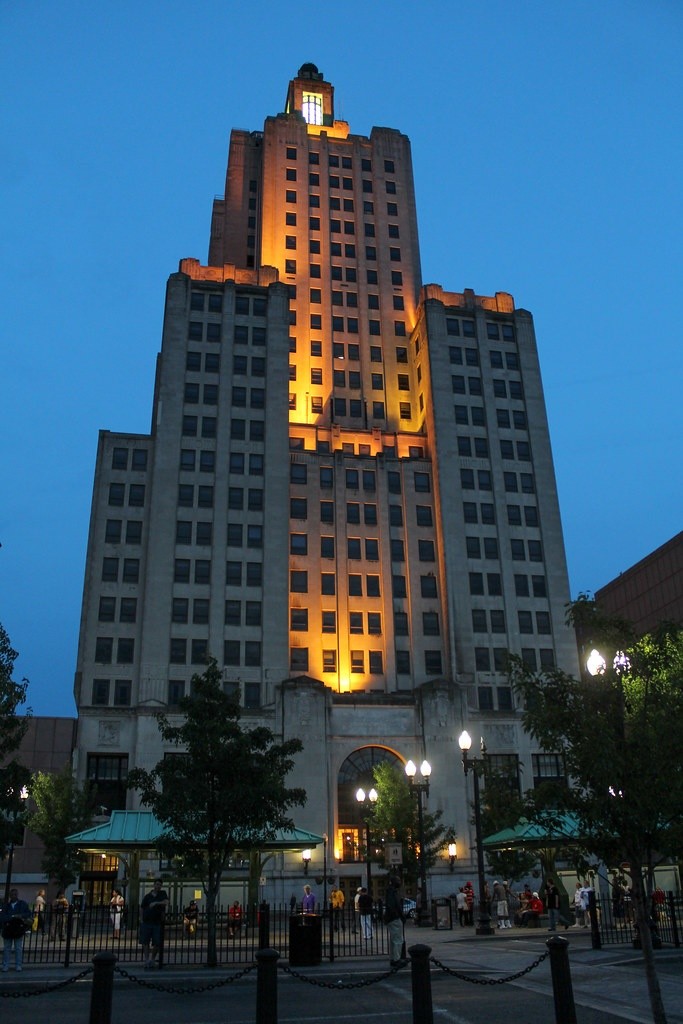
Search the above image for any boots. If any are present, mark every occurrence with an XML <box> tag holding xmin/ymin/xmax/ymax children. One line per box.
<box><xmin>572</xmin><ymin>918</ymin><xmax>580</xmax><ymax>928</ymax></box>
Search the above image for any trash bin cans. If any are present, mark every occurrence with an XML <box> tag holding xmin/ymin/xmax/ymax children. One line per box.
<box><xmin>432</xmin><ymin>897</ymin><xmax>453</xmax><ymax>930</ymax></box>
<box><xmin>290</xmin><ymin>914</ymin><xmax>322</xmax><ymax>966</ymax></box>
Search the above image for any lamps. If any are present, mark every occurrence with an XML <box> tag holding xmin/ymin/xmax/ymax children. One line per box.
<box><xmin>447</xmin><ymin>835</ymin><xmax>457</xmax><ymax>872</ymax></box>
<box><xmin>301</xmin><ymin>849</ymin><xmax>312</xmax><ymax>874</ymax></box>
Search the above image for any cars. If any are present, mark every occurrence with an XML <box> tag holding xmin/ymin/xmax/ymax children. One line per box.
<box><xmin>372</xmin><ymin>896</ymin><xmax>416</xmax><ymax>919</ymax></box>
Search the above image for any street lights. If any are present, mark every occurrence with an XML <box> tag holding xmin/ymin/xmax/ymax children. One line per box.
<box><xmin>586</xmin><ymin>646</ymin><xmax>670</xmax><ymax>1024</ymax></box>
<box><xmin>459</xmin><ymin>730</ymin><xmax>496</xmax><ymax>936</ymax></box>
<box><xmin>356</xmin><ymin>787</ymin><xmax>378</xmax><ymax>896</ymax></box>
<box><xmin>323</xmin><ymin>833</ymin><xmax>328</xmax><ymax>910</ymax></box>
<box><xmin>405</xmin><ymin>759</ymin><xmax>432</xmax><ymax>926</ymax></box>
<box><xmin>5</xmin><ymin>785</ymin><xmax>29</xmax><ymax>905</ymax></box>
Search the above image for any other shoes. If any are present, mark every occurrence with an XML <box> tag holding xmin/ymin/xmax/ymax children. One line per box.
<box><xmin>15</xmin><ymin>964</ymin><xmax>23</xmax><ymax>971</ymax></box>
<box><xmin>143</xmin><ymin>960</ymin><xmax>156</xmax><ymax>969</ymax></box>
<box><xmin>2</xmin><ymin>965</ymin><xmax>9</xmax><ymax>971</ymax></box>
<box><xmin>51</xmin><ymin>937</ymin><xmax>65</xmax><ymax>941</ymax></box>
<box><xmin>565</xmin><ymin>921</ymin><xmax>570</xmax><ymax>929</ymax></box>
<box><xmin>390</xmin><ymin>959</ymin><xmax>407</xmax><ymax>968</ymax></box>
<box><xmin>496</xmin><ymin>925</ymin><xmax>512</xmax><ymax>929</ymax></box>
<box><xmin>548</xmin><ymin>928</ymin><xmax>557</xmax><ymax>931</ymax></box>
<box><xmin>110</xmin><ymin>935</ymin><xmax>120</xmax><ymax>939</ymax></box>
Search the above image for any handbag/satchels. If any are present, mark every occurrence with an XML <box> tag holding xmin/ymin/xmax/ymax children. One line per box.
<box><xmin>190</xmin><ymin>923</ymin><xmax>197</xmax><ymax>936</ymax></box>
<box><xmin>462</xmin><ymin>903</ymin><xmax>469</xmax><ymax>911</ymax></box>
<box><xmin>2</xmin><ymin>913</ymin><xmax>43</xmax><ymax>939</ymax></box>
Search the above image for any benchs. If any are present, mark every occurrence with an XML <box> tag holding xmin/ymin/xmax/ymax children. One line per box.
<box><xmin>527</xmin><ymin>914</ymin><xmax>550</xmax><ymax>928</ymax></box>
<box><xmin>165</xmin><ymin>922</ymin><xmax>246</xmax><ymax>938</ymax></box>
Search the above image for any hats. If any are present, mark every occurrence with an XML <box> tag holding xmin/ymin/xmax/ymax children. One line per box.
<box><xmin>467</xmin><ymin>881</ymin><xmax>472</xmax><ymax>886</ymax></box>
<box><xmin>357</xmin><ymin>887</ymin><xmax>362</xmax><ymax>891</ymax></box>
<box><xmin>190</xmin><ymin>900</ymin><xmax>196</xmax><ymax>905</ymax></box>
<box><xmin>492</xmin><ymin>880</ymin><xmax>499</xmax><ymax>885</ymax></box>
<box><xmin>503</xmin><ymin>881</ymin><xmax>508</xmax><ymax>886</ymax></box>
<box><xmin>533</xmin><ymin>892</ymin><xmax>540</xmax><ymax>899</ymax></box>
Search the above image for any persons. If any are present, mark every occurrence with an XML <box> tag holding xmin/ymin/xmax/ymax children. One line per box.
<box><xmin>139</xmin><ymin>879</ymin><xmax>169</xmax><ymax>968</ymax></box>
<box><xmin>33</xmin><ymin>879</ymin><xmax>666</xmax><ymax>940</ymax></box>
<box><xmin>385</xmin><ymin>876</ymin><xmax>407</xmax><ymax>967</ymax></box>
<box><xmin>0</xmin><ymin>889</ymin><xmax>31</xmax><ymax>972</ymax></box>
